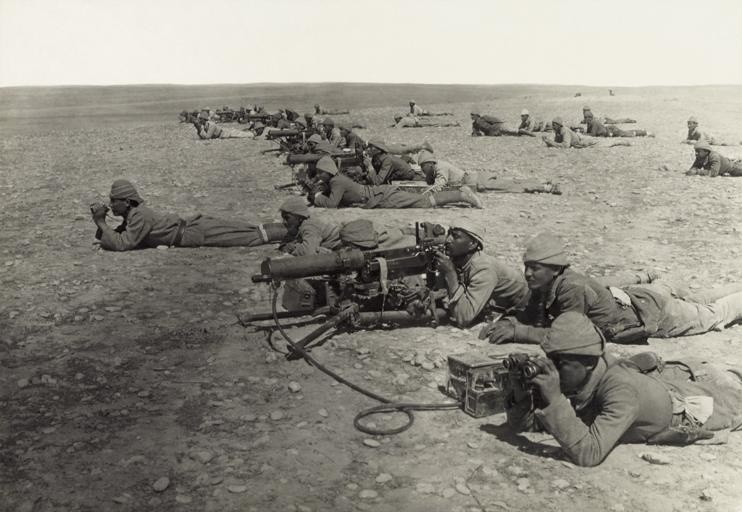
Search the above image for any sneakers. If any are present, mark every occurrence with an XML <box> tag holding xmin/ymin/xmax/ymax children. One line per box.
<box><xmin>543</xmin><ymin>176</ymin><xmax>563</xmax><ymax>195</ymax></box>
<box><xmin>459</xmin><ymin>185</ymin><xmax>484</xmax><ymax>209</ymax></box>
<box><xmin>646</xmin><ymin>269</ymin><xmax>662</xmax><ymax>283</ymax></box>
<box><xmin>626</xmin><ymin>118</ymin><xmax>637</xmax><ymax>124</ymax></box>
<box><xmin>422</xmin><ymin>138</ymin><xmax>434</xmax><ymax>153</ymax></box>
<box><xmin>643</xmin><ymin>128</ymin><xmax>655</xmax><ymax>139</ymax></box>
<box><xmin>624</xmin><ymin>138</ymin><xmax>633</xmax><ymax>146</ymax></box>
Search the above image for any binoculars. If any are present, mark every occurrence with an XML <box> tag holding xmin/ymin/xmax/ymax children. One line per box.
<box><xmin>89</xmin><ymin>205</ymin><xmax>110</xmax><ymax>214</ymax></box>
<box><xmin>503</xmin><ymin>352</ymin><xmax>542</xmax><ymax>379</ymax></box>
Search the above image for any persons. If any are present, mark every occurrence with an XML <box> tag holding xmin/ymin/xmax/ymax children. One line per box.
<box><xmin>89</xmin><ymin>177</ymin><xmax>289</xmax><ymax>253</ymax></box>
<box><xmin>431</xmin><ymin>215</ymin><xmax>707</xmax><ymax>329</ymax></box>
<box><xmin>480</xmin><ymin>233</ymin><xmax>742</xmax><ymax>344</ymax></box>
<box><xmin>181</xmin><ymin>100</ymin><xmax>482</xmax><ymax>210</ymax></box>
<box><xmin>279</xmin><ymin>196</ymin><xmax>426</xmax><ymax>256</ymax></box>
<box><xmin>471</xmin><ymin>106</ymin><xmax>656</xmax><ymax>149</ymax></box>
<box><xmin>684</xmin><ymin>139</ymin><xmax>742</xmax><ymax>177</ymax></box>
<box><xmin>504</xmin><ymin>310</ymin><xmax>741</xmax><ymax>465</ymax></box>
<box><xmin>683</xmin><ymin>117</ymin><xmax>741</xmax><ymax>147</ymax></box>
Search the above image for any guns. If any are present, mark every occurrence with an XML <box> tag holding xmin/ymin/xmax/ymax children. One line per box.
<box><xmin>258</xmin><ymin>142</ymin><xmax>294</xmax><ymax>152</ymax></box>
<box><xmin>216</xmin><ymin>109</ymin><xmax>320</xmax><ymax>142</ymax></box>
<box><xmin>236</xmin><ymin>222</ymin><xmax>447</xmax><ymax>362</ymax></box>
<box><xmin>283</xmin><ymin>151</ymin><xmax>367</xmax><ymax>183</ymax></box>
<box><xmin>274</xmin><ymin>175</ymin><xmax>320</xmax><ymax>190</ymax></box>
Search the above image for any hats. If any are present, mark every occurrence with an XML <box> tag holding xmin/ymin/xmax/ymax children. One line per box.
<box><xmin>470</xmin><ymin>110</ymin><xmax>480</xmax><ymax>117</ymax></box>
<box><xmin>418</xmin><ymin>150</ymin><xmax>437</xmax><ymax>165</ymax></box>
<box><xmin>109</xmin><ymin>179</ymin><xmax>143</xmax><ymax>204</ymax></box>
<box><xmin>216</xmin><ymin>104</ymin><xmax>294</xmax><ymax>120</ymax></box>
<box><xmin>522</xmin><ymin>232</ymin><xmax>570</xmax><ymax>267</ymax></box>
<box><xmin>686</xmin><ymin>115</ymin><xmax>712</xmax><ymax>152</ymax></box>
<box><xmin>278</xmin><ymin>196</ymin><xmax>310</xmax><ymax>218</ymax></box>
<box><xmin>448</xmin><ymin>214</ymin><xmax>486</xmax><ymax>250</ymax></box>
<box><xmin>182</xmin><ymin>107</ymin><xmax>210</xmax><ymax>121</ymax></box>
<box><xmin>521</xmin><ymin>105</ymin><xmax>594</xmax><ymax>126</ymax></box>
<box><xmin>394</xmin><ymin>99</ymin><xmax>416</xmax><ymax>119</ymax></box>
<box><xmin>540</xmin><ymin>312</ymin><xmax>606</xmax><ymax>357</ymax></box>
<box><xmin>339</xmin><ymin>219</ymin><xmax>379</xmax><ymax>249</ymax></box>
<box><xmin>304</xmin><ymin>113</ymin><xmax>389</xmax><ymax>176</ymax></box>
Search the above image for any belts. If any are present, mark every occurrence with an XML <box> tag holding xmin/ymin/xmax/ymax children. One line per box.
<box><xmin>215</xmin><ymin>128</ymin><xmax>223</xmax><ymax>138</ymax></box>
<box><xmin>173</xmin><ymin>219</ymin><xmax>186</xmax><ymax>247</ymax></box>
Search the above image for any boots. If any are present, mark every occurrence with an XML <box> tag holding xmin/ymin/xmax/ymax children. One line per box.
<box><xmin>518</xmin><ymin>128</ymin><xmax>537</xmax><ymax>137</ymax></box>
